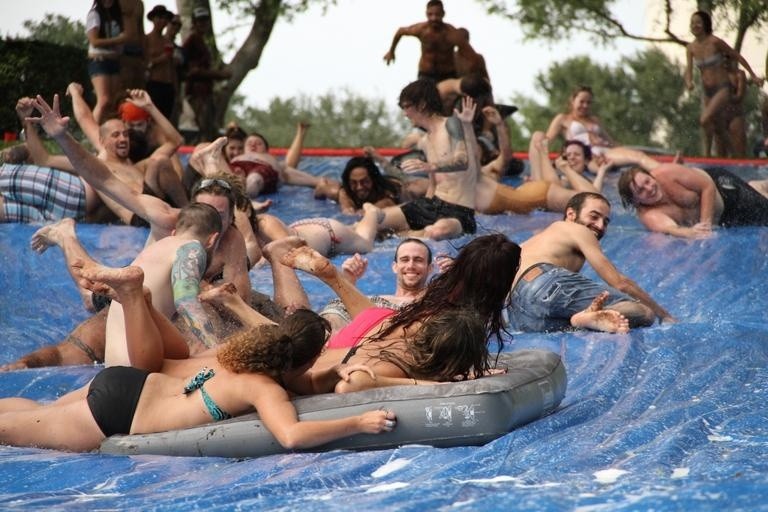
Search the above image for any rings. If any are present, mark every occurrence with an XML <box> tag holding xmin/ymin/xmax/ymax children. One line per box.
<box><xmin>385</xmin><ymin>412</ymin><xmax>388</xmax><ymax>419</ymax></box>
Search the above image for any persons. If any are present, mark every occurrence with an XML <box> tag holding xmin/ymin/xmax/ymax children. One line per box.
<box><xmin>1</xmin><ymin>2</ymin><xmax>768</xmax><ymax>396</ymax></box>
<box><xmin>0</xmin><ymin>306</ymin><xmax>396</xmax><ymax>453</ymax></box>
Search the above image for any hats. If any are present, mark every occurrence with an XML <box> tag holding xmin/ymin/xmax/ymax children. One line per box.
<box><xmin>191</xmin><ymin>7</ymin><xmax>210</xmax><ymax>21</ymax></box>
<box><xmin>146</xmin><ymin>5</ymin><xmax>174</xmax><ymax>21</ymax></box>
